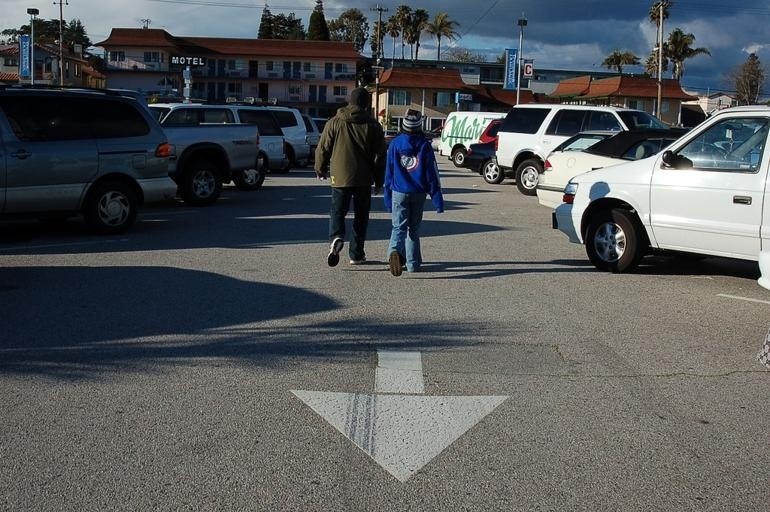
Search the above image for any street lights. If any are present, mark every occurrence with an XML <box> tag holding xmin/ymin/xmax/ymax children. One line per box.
<box><xmin>376</xmin><ymin>58</ymin><xmax>382</xmax><ymax>122</ymax></box>
<box><xmin>27</xmin><ymin>8</ymin><xmax>39</xmax><ymax>85</ymax></box>
<box><xmin>652</xmin><ymin>46</ymin><xmax>663</xmax><ymax>119</ymax></box>
<box><xmin>53</xmin><ymin>40</ymin><xmax>64</xmax><ymax>84</ymax></box>
<box><xmin>517</xmin><ymin>11</ymin><xmax>528</xmax><ymax>105</ymax></box>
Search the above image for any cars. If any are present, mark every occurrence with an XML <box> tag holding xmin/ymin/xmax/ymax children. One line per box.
<box><xmin>548</xmin><ymin>103</ymin><xmax>770</xmax><ymax>275</ymax></box>
<box><xmin>424</xmin><ymin>100</ymin><xmax>696</xmax><ymax>211</ymax></box>
<box><xmin>384</xmin><ymin>130</ymin><xmax>400</xmax><ymax>147</ymax></box>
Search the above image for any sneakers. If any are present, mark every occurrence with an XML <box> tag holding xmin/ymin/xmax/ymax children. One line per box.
<box><xmin>327</xmin><ymin>238</ymin><xmax>344</xmax><ymax>267</ymax></box>
<box><xmin>349</xmin><ymin>257</ymin><xmax>366</xmax><ymax>265</ymax></box>
<box><xmin>389</xmin><ymin>251</ymin><xmax>402</xmax><ymax>276</ymax></box>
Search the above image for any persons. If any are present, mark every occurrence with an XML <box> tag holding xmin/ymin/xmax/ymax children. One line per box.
<box><xmin>314</xmin><ymin>87</ymin><xmax>387</xmax><ymax>267</ymax></box>
<box><xmin>384</xmin><ymin>109</ymin><xmax>443</xmax><ymax>276</ymax></box>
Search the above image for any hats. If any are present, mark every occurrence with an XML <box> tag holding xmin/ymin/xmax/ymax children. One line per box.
<box><xmin>351</xmin><ymin>87</ymin><xmax>369</xmax><ymax>107</ymax></box>
<box><xmin>402</xmin><ymin>108</ymin><xmax>422</xmax><ymax>132</ymax></box>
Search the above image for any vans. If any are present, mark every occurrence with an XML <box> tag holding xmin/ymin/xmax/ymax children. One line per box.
<box><xmin>145</xmin><ymin>101</ymin><xmax>290</xmax><ymax>192</ymax></box>
<box><xmin>311</xmin><ymin>117</ymin><xmax>328</xmax><ymax>138</ymax></box>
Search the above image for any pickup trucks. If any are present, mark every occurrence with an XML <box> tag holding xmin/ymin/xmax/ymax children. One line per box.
<box><xmin>160</xmin><ymin>124</ymin><xmax>260</xmax><ymax>207</ymax></box>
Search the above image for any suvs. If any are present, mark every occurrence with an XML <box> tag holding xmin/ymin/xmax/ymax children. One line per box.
<box><xmin>301</xmin><ymin>113</ymin><xmax>320</xmax><ymax>154</ymax></box>
<box><xmin>0</xmin><ymin>83</ymin><xmax>180</xmax><ymax>236</ymax></box>
<box><xmin>226</xmin><ymin>97</ymin><xmax>311</xmax><ymax>173</ymax></box>
